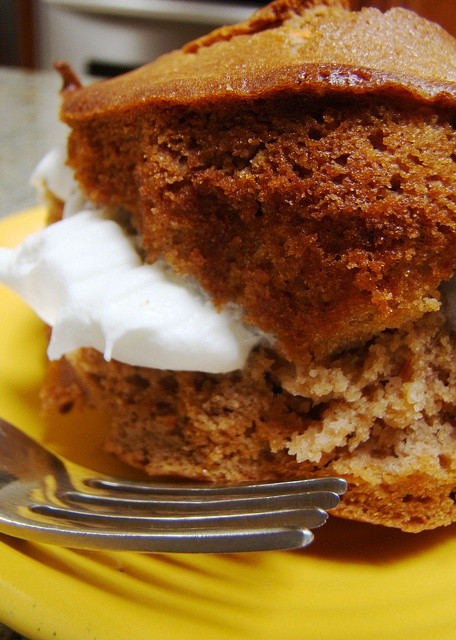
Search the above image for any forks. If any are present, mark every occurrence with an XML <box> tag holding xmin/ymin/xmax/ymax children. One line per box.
<box><xmin>2</xmin><ymin>417</ymin><xmax>350</xmax><ymax>551</ymax></box>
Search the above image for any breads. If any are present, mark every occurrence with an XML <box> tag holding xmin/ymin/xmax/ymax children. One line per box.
<box><xmin>41</xmin><ymin>2</ymin><xmax>455</xmax><ymax>534</ymax></box>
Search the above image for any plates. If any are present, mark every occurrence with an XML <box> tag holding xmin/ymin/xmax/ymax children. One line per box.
<box><xmin>2</xmin><ymin>205</ymin><xmax>455</xmax><ymax>640</ymax></box>
